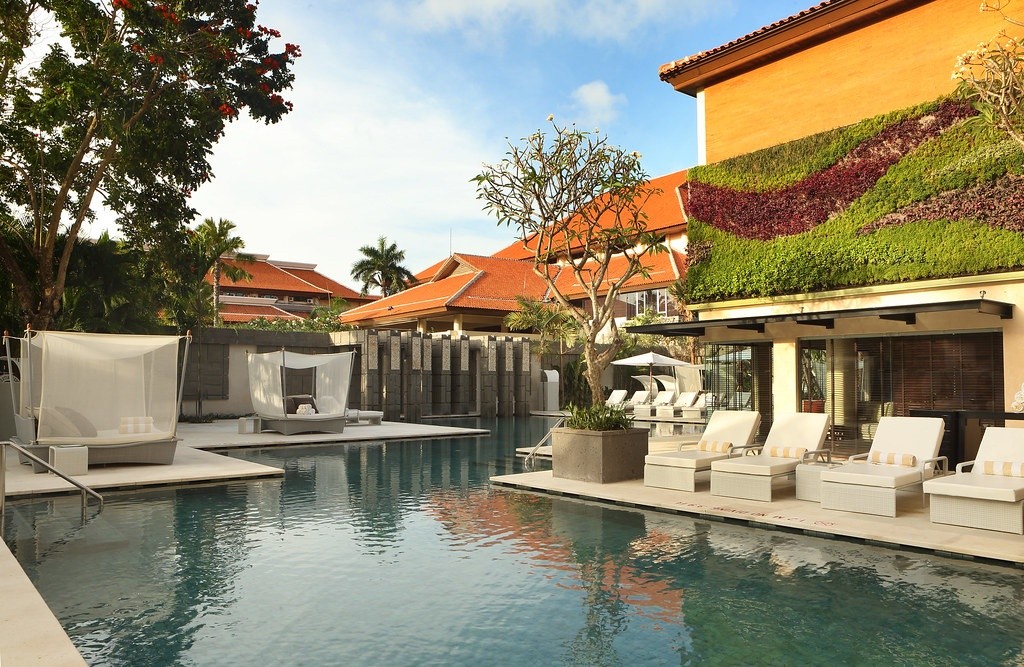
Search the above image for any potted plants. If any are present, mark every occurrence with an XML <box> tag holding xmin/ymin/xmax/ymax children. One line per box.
<box><xmin>544</xmin><ymin>401</ymin><xmax>650</xmax><ymax>483</ymax></box>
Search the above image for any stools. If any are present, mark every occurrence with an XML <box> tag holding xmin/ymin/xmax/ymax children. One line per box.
<box><xmin>47</xmin><ymin>445</ymin><xmax>90</xmax><ymax>476</ymax></box>
<box><xmin>237</xmin><ymin>417</ymin><xmax>262</xmax><ymax>434</ymax></box>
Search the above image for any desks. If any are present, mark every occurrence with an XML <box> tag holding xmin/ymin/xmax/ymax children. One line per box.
<box><xmin>794</xmin><ymin>459</ymin><xmax>843</xmax><ymax>501</ymax></box>
<box><xmin>345</xmin><ymin>410</ymin><xmax>384</xmax><ymax>425</ymax></box>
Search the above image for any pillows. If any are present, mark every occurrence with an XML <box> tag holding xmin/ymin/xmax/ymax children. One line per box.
<box><xmin>282</xmin><ymin>397</ymin><xmax>319</xmax><ymax>414</ymax></box>
<box><xmin>25</xmin><ymin>406</ymin><xmax>98</xmax><ymax>438</ymax></box>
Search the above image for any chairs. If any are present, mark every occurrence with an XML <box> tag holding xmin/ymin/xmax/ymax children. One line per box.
<box><xmin>643</xmin><ymin>411</ymin><xmax>762</xmax><ymax>493</ymax></box>
<box><xmin>922</xmin><ymin>426</ymin><xmax>1024</xmax><ymax>534</ymax></box>
<box><xmin>707</xmin><ymin>412</ymin><xmax>831</xmax><ymax>503</ymax></box>
<box><xmin>821</xmin><ymin>415</ymin><xmax>947</xmax><ymax>521</ymax></box>
<box><xmin>604</xmin><ymin>390</ymin><xmax>751</xmax><ymax>419</ymax></box>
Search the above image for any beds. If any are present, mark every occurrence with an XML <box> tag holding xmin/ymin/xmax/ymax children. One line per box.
<box><xmin>4</xmin><ymin>323</ymin><xmax>191</xmax><ymax>473</ymax></box>
<box><xmin>244</xmin><ymin>348</ymin><xmax>357</xmax><ymax>436</ymax></box>
